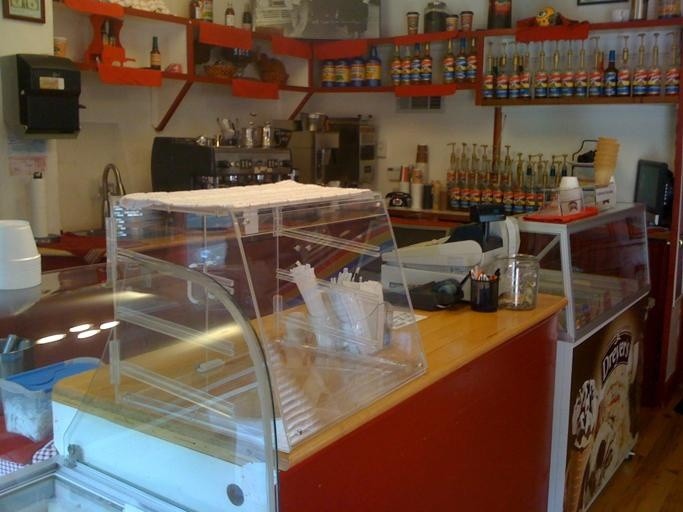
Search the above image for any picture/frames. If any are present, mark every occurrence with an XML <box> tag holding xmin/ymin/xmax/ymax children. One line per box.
<box><xmin>1</xmin><ymin>0</ymin><xmax>46</xmax><ymax>24</ymax></box>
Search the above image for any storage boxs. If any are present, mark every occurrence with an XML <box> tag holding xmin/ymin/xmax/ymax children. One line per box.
<box><xmin>0</xmin><ymin>356</ymin><xmax>106</xmax><ymax>442</ymax></box>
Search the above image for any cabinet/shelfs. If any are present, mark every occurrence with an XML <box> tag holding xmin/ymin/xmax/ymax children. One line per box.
<box><xmin>152</xmin><ymin>133</ymin><xmax>296</xmax><ymax>195</ymax></box>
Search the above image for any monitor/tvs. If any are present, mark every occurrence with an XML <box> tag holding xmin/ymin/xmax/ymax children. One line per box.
<box><xmin>633</xmin><ymin>160</ymin><xmax>674</xmax><ymax>214</ymax></box>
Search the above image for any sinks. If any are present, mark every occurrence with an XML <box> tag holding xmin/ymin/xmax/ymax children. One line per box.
<box><xmin>85</xmin><ymin>226</ymin><xmax>185</xmax><ymax>241</ymax></box>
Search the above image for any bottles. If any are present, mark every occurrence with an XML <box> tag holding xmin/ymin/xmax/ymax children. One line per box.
<box><xmin>202</xmin><ymin>0</ymin><xmax>213</xmax><ymax>22</ymax></box>
<box><xmin>604</xmin><ymin>50</ymin><xmax>617</xmax><ymax>96</ymax></box>
<box><xmin>466</xmin><ymin>37</ymin><xmax>477</xmax><ymax>82</ymax></box>
<box><xmin>633</xmin><ymin>46</ymin><xmax>649</xmax><ymax>95</ymax></box>
<box><xmin>365</xmin><ymin>45</ymin><xmax>380</xmax><ymax>87</ymax></box>
<box><xmin>666</xmin><ymin>44</ymin><xmax>680</xmax><ymax>94</ymax></box>
<box><xmin>456</xmin><ymin>36</ymin><xmax>468</xmax><ymax>81</ymax></box>
<box><xmin>576</xmin><ymin>50</ymin><xmax>588</xmax><ymax>96</ymax></box>
<box><xmin>471</xmin><ymin>170</ymin><xmax>480</xmax><ymax>207</ymax></box>
<box><xmin>493</xmin><ymin>170</ymin><xmax>503</xmax><ymax>207</ymax></box>
<box><xmin>411</xmin><ymin>43</ymin><xmax>421</xmax><ymax>85</ymax></box>
<box><xmin>485</xmin><ymin>54</ymin><xmax>495</xmax><ymax>98</ymax></box>
<box><xmin>513</xmin><ymin>171</ymin><xmax>525</xmax><ymax>213</ymax></box>
<box><xmin>526</xmin><ymin>172</ymin><xmax>536</xmax><ymax>211</ymax></box>
<box><xmin>402</xmin><ymin>45</ymin><xmax>410</xmax><ymax>85</ymax></box>
<box><xmin>648</xmin><ymin>46</ymin><xmax>662</xmax><ymax>95</ymax></box>
<box><xmin>562</xmin><ymin>50</ymin><xmax>575</xmax><ymax>96</ymax></box>
<box><xmin>490</xmin><ymin>252</ymin><xmax>540</xmax><ymax>310</ymax></box>
<box><xmin>511</xmin><ymin>54</ymin><xmax>521</xmax><ymax>98</ymax></box>
<box><xmin>262</xmin><ymin>120</ymin><xmax>272</xmax><ymax>149</ymax></box>
<box><xmin>451</xmin><ymin>169</ymin><xmax>461</xmax><ymax>210</ymax></box>
<box><xmin>534</xmin><ymin>49</ymin><xmax>548</xmax><ymax>97</ymax></box>
<box><xmin>188</xmin><ymin>0</ymin><xmax>200</xmax><ymax>19</ymax></box>
<box><xmin>421</xmin><ymin>41</ymin><xmax>433</xmax><ymax>83</ymax></box>
<box><xmin>483</xmin><ymin>172</ymin><xmax>492</xmax><ymax>207</ymax></box>
<box><xmin>470</xmin><ymin>153</ymin><xmax>479</xmax><ymax>185</ymax></box>
<box><xmin>538</xmin><ymin>172</ymin><xmax>549</xmax><ymax>207</ymax></box>
<box><xmin>503</xmin><ymin>169</ymin><xmax>513</xmax><ymax>213</ymax></box>
<box><xmin>320</xmin><ymin>59</ymin><xmax>336</xmax><ymax>88</ymax></box>
<box><xmin>242</xmin><ymin>4</ymin><xmax>250</xmax><ymax>29</ymax></box>
<box><xmin>618</xmin><ymin>47</ymin><xmax>631</xmax><ymax>96</ymax></box>
<box><xmin>336</xmin><ymin>60</ymin><xmax>350</xmax><ymax>88</ymax></box>
<box><xmin>549</xmin><ymin>165</ymin><xmax>556</xmax><ymax>187</ymax></box>
<box><xmin>150</xmin><ymin>36</ymin><xmax>161</xmax><ymax>70</ymax></box>
<box><xmin>460</xmin><ymin>168</ymin><xmax>470</xmax><ymax>209</ymax></box>
<box><xmin>549</xmin><ymin>50</ymin><xmax>561</xmax><ymax>97</ymax></box>
<box><xmin>526</xmin><ymin>165</ymin><xmax>533</xmax><ymax>187</ymax></box>
<box><xmin>350</xmin><ymin>59</ymin><xmax>365</xmax><ymax>86</ymax></box>
<box><xmin>561</xmin><ymin>165</ymin><xmax>568</xmax><ymax>177</ymax></box>
<box><xmin>441</xmin><ymin>38</ymin><xmax>456</xmax><ymax>82</ymax></box>
<box><xmin>589</xmin><ymin>49</ymin><xmax>602</xmax><ymax>95</ymax></box>
<box><xmin>522</xmin><ymin>52</ymin><xmax>533</xmax><ymax>98</ymax></box>
<box><xmin>390</xmin><ymin>46</ymin><xmax>400</xmax><ymax>86</ymax></box>
<box><xmin>503</xmin><ymin>156</ymin><xmax>512</xmax><ymax>184</ymax></box>
<box><xmin>447</xmin><ymin>152</ymin><xmax>457</xmax><ymax>184</ymax></box>
<box><xmin>225</xmin><ymin>2</ymin><xmax>236</xmax><ymax>25</ymax></box>
<box><xmin>459</xmin><ymin>153</ymin><xmax>469</xmax><ymax>182</ymax></box>
<box><xmin>497</xmin><ymin>55</ymin><xmax>509</xmax><ymax>98</ymax></box>
<box><xmin>480</xmin><ymin>155</ymin><xmax>489</xmax><ymax>185</ymax></box>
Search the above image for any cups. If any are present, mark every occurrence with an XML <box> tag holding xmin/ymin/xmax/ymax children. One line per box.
<box><xmin>410</xmin><ymin>169</ymin><xmax>424</xmax><ymax>211</ymax></box>
<box><xmin>416</xmin><ymin>143</ymin><xmax>428</xmax><ymax>184</ymax></box>
<box><xmin>460</xmin><ymin>10</ymin><xmax>474</xmax><ymax>32</ymax></box>
<box><xmin>53</xmin><ymin>36</ymin><xmax>68</xmax><ymax>58</ymax></box>
<box><xmin>399</xmin><ymin>164</ymin><xmax>410</xmax><ymax>194</ymax></box>
<box><xmin>445</xmin><ymin>14</ymin><xmax>459</xmax><ymax>33</ymax></box>
<box><xmin>406</xmin><ymin>11</ymin><xmax>420</xmax><ymax>36</ymax></box>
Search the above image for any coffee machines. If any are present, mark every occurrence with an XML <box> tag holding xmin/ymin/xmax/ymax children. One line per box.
<box><xmin>150</xmin><ymin>137</ymin><xmax>221</xmax><ymax>231</ymax></box>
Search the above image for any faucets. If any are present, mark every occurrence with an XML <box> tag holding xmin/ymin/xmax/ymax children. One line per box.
<box><xmin>99</xmin><ymin>165</ymin><xmax>127</xmax><ymax>227</ymax></box>
